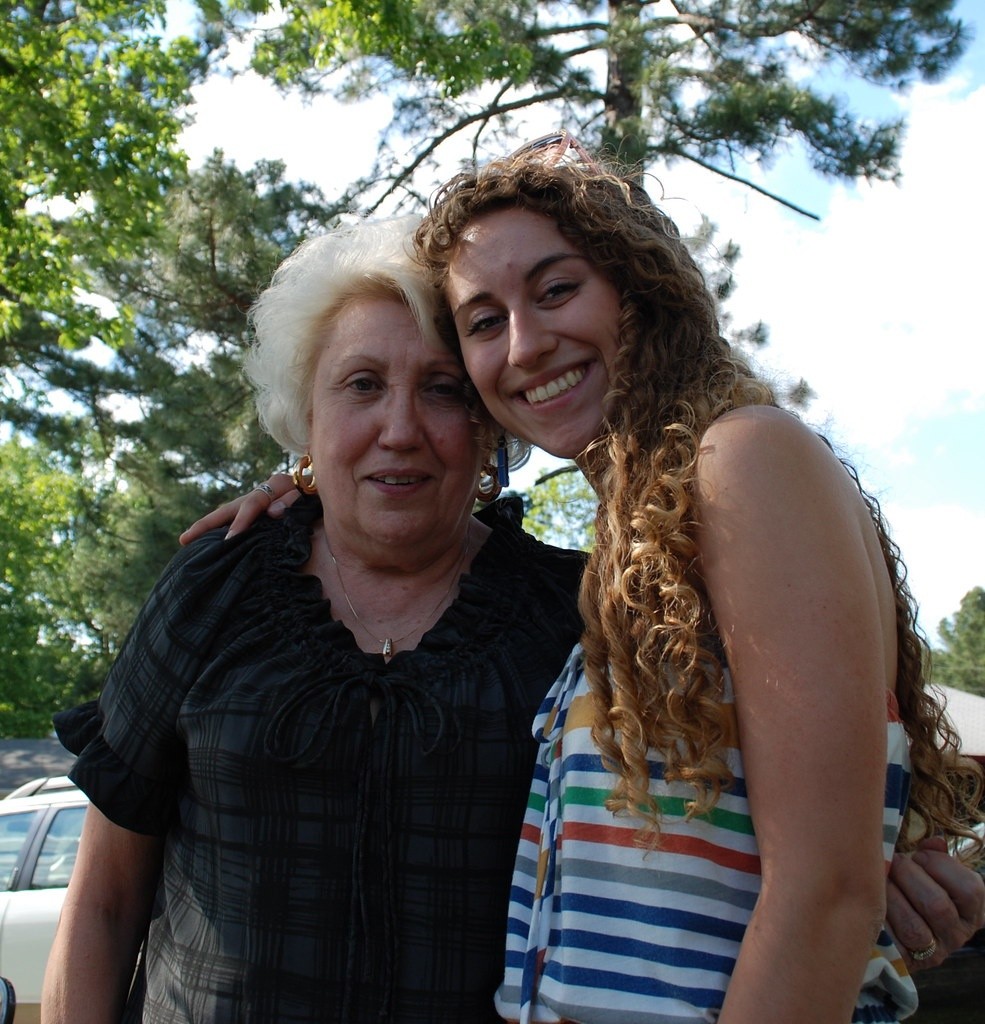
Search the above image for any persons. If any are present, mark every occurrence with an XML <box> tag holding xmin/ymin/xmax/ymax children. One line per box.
<box><xmin>41</xmin><ymin>128</ymin><xmax>985</xmax><ymax>1024</ymax></box>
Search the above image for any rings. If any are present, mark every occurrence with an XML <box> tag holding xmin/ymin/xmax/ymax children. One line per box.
<box><xmin>907</xmin><ymin>938</ymin><xmax>936</xmax><ymax>961</ymax></box>
<box><xmin>254</xmin><ymin>482</ymin><xmax>276</xmax><ymax>502</ymax></box>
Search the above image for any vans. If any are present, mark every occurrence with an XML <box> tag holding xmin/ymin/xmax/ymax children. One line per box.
<box><xmin>0</xmin><ymin>776</ymin><xmax>93</xmax><ymax>1024</ymax></box>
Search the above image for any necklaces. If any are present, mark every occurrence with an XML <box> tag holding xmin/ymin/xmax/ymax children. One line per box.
<box><xmin>321</xmin><ymin>518</ymin><xmax>471</xmax><ymax>656</ymax></box>
